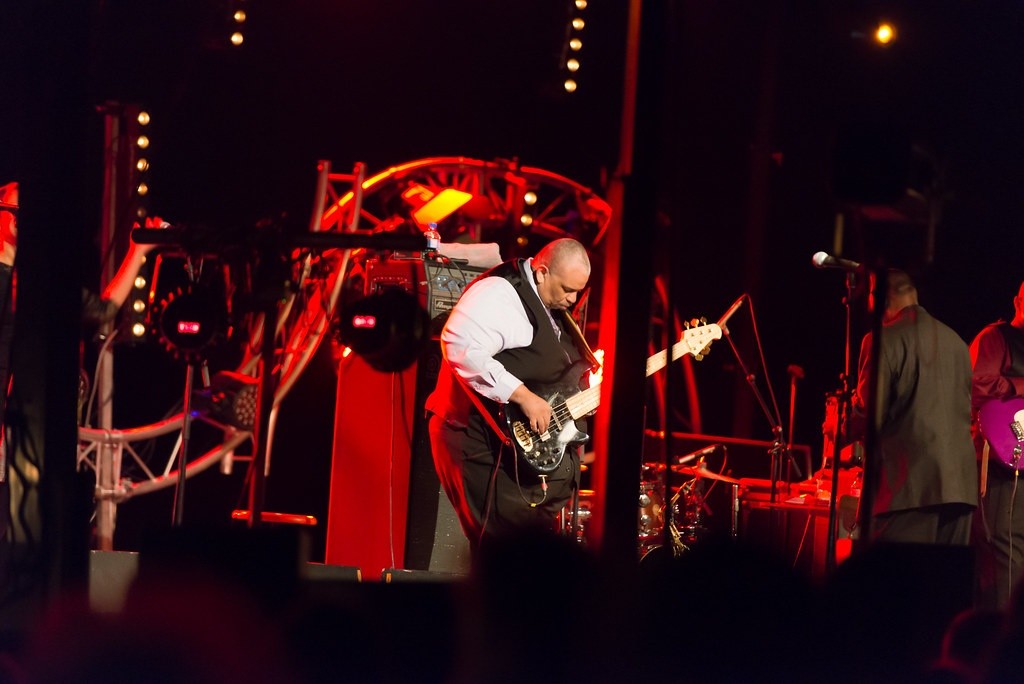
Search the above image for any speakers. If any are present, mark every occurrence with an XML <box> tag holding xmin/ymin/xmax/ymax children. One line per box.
<box><xmin>325</xmin><ymin>255</ymin><xmax>487</xmax><ymax>577</ymax></box>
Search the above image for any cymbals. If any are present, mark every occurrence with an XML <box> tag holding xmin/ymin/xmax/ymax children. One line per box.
<box><xmin>641</xmin><ymin>460</ymin><xmax>738</xmax><ymax>486</ymax></box>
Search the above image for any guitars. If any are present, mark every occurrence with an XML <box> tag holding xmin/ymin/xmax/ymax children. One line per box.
<box><xmin>977</xmin><ymin>394</ymin><xmax>1024</xmax><ymax>471</ymax></box>
<box><xmin>497</xmin><ymin>313</ymin><xmax>723</xmax><ymax>474</ymax></box>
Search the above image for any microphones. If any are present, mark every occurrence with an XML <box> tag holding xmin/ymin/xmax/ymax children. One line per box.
<box><xmin>716</xmin><ymin>294</ymin><xmax>746</xmax><ymax>328</ymax></box>
<box><xmin>812</xmin><ymin>251</ymin><xmax>864</xmax><ymax>272</ymax></box>
<box><xmin>679</xmin><ymin>444</ymin><xmax>723</xmax><ymax>464</ymax></box>
<box><xmin>788</xmin><ymin>365</ymin><xmax>804</xmax><ymax>379</ymax></box>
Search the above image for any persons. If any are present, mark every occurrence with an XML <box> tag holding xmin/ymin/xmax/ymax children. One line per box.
<box><xmin>966</xmin><ymin>279</ymin><xmax>1024</xmax><ymax>611</ymax></box>
<box><xmin>822</xmin><ymin>267</ymin><xmax>978</xmax><ymax>544</ymax></box>
<box><xmin>424</xmin><ymin>241</ymin><xmax>602</xmax><ymax>541</ymax></box>
<box><xmin>102</xmin><ymin>216</ymin><xmax>167</xmax><ymax>323</ymax></box>
<box><xmin>36</xmin><ymin>523</ymin><xmax>1023</xmax><ymax>684</ymax></box>
